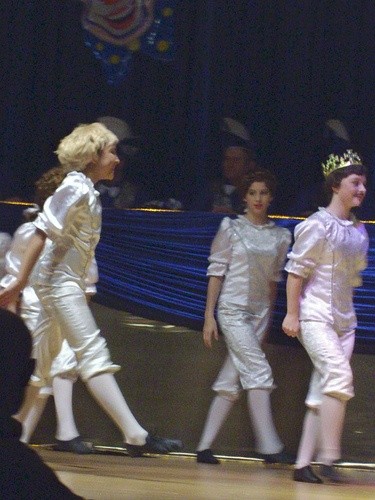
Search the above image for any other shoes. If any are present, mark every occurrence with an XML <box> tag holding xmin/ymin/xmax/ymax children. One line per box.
<box><xmin>56</xmin><ymin>436</ymin><xmax>96</xmax><ymax>454</ymax></box>
<box><xmin>123</xmin><ymin>432</ymin><xmax>186</xmax><ymax>458</ymax></box>
<box><xmin>196</xmin><ymin>448</ymin><xmax>220</xmax><ymax>464</ymax></box>
<box><xmin>294</xmin><ymin>463</ymin><xmax>346</xmax><ymax>483</ymax></box>
<box><xmin>262</xmin><ymin>452</ymin><xmax>296</xmax><ymax>464</ymax></box>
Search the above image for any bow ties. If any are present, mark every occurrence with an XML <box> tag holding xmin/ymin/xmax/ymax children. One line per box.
<box><xmin>98</xmin><ymin>184</ymin><xmax>120</xmax><ymax>196</ymax></box>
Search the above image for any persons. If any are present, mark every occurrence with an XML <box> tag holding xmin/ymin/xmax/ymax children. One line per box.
<box><xmin>204</xmin><ymin>144</ymin><xmax>255</xmax><ymax>213</ymax></box>
<box><xmin>195</xmin><ymin>170</ymin><xmax>293</xmax><ymax>465</ymax></box>
<box><xmin>282</xmin><ymin>149</ymin><xmax>370</xmax><ymax>483</ymax></box>
<box><xmin>0</xmin><ymin>121</ymin><xmax>185</xmax><ymax>500</ymax></box>
